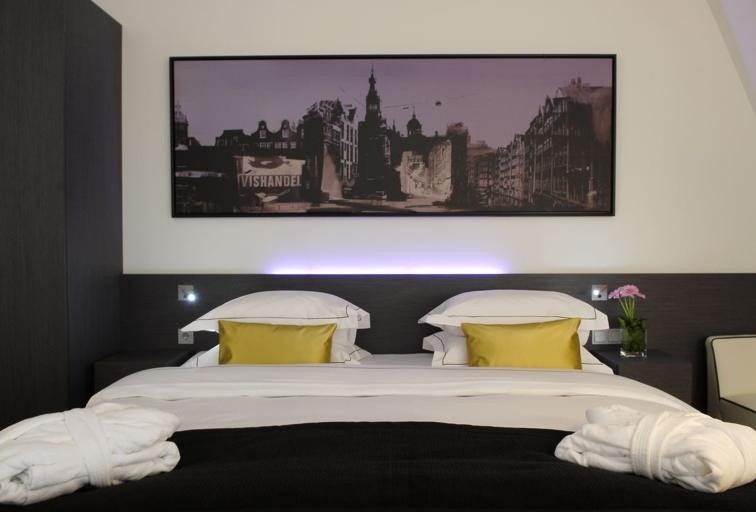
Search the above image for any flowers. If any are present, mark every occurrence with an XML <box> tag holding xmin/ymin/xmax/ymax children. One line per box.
<box><xmin>609</xmin><ymin>284</ymin><xmax>647</xmax><ymax>353</ymax></box>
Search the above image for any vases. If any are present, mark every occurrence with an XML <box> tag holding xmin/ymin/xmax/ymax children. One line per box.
<box><xmin>618</xmin><ymin>329</ymin><xmax>647</xmax><ymax>361</ymax></box>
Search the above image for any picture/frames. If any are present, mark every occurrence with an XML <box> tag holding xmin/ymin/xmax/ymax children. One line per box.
<box><xmin>169</xmin><ymin>54</ymin><xmax>616</xmax><ymax>218</ymax></box>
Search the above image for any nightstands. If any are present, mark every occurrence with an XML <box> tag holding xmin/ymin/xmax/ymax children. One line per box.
<box><xmin>94</xmin><ymin>352</ymin><xmax>191</xmax><ymax>392</ymax></box>
<box><xmin>590</xmin><ymin>349</ymin><xmax>694</xmax><ymax>407</ymax></box>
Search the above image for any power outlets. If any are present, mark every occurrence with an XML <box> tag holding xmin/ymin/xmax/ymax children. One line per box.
<box><xmin>178</xmin><ymin>285</ymin><xmax>194</xmax><ymax>301</ymax></box>
<box><xmin>177</xmin><ymin>329</ymin><xmax>193</xmax><ymax>345</ymax></box>
<box><xmin>591</xmin><ymin>284</ymin><xmax>608</xmax><ymax>301</ymax></box>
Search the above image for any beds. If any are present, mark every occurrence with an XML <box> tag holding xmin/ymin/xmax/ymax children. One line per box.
<box><xmin>0</xmin><ymin>353</ymin><xmax>756</xmax><ymax>512</ymax></box>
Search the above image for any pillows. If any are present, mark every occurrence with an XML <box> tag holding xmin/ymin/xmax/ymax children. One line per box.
<box><xmin>423</xmin><ymin>331</ymin><xmax>614</xmax><ymax>376</ymax></box>
<box><xmin>218</xmin><ymin>320</ymin><xmax>337</xmax><ymax>364</ymax></box>
<box><xmin>418</xmin><ymin>290</ymin><xmax>609</xmax><ymax>336</ymax></box>
<box><xmin>179</xmin><ymin>290</ymin><xmax>371</xmax><ymax>344</ymax></box>
<box><xmin>461</xmin><ymin>318</ymin><xmax>583</xmax><ymax>370</ymax></box>
<box><xmin>198</xmin><ymin>341</ymin><xmax>374</xmax><ymax>363</ymax></box>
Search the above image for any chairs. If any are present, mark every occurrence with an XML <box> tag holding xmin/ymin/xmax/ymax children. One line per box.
<box><xmin>705</xmin><ymin>334</ymin><xmax>756</xmax><ymax>430</ymax></box>
